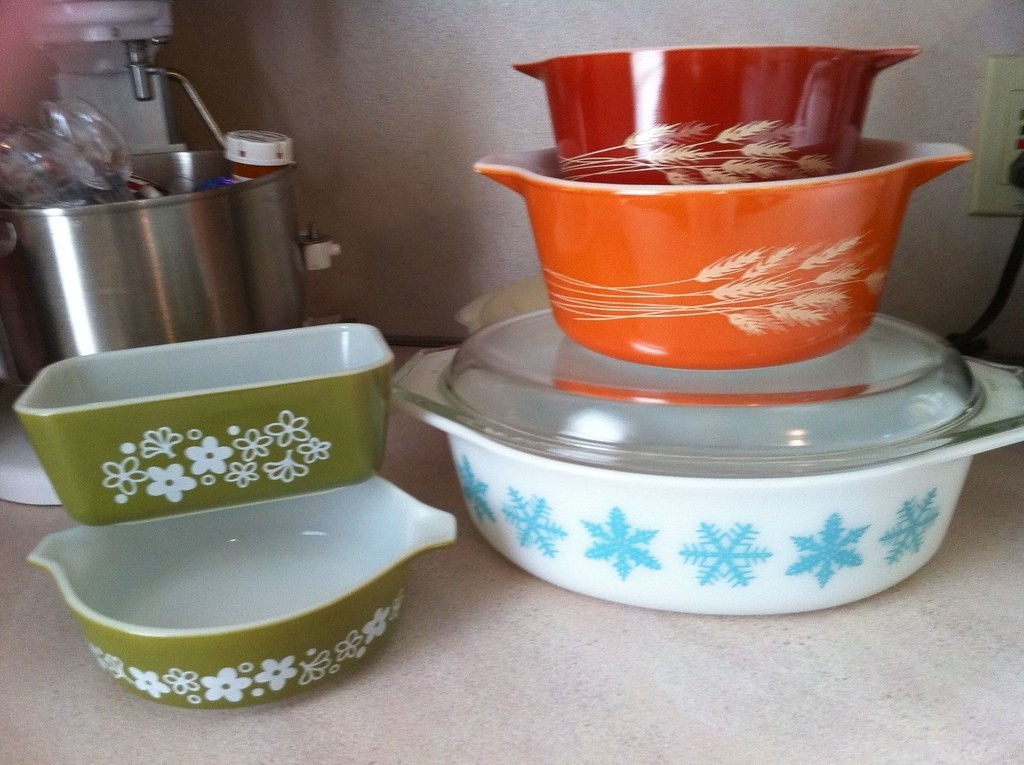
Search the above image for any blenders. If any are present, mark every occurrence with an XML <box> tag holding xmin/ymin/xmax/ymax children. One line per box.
<box><xmin>0</xmin><ymin>0</ymin><xmax>315</xmax><ymax>508</ymax></box>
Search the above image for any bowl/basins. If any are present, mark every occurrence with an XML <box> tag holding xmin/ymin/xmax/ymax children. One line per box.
<box><xmin>512</xmin><ymin>45</ymin><xmax>924</xmax><ymax>189</ymax></box>
<box><xmin>13</xmin><ymin>323</ymin><xmax>392</xmax><ymax>528</ymax></box>
<box><xmin>471</xmin><ymin>136</ymin><xmax>976</xmax><ymax>371</ymax></box>
<box><xmin>24</xmin><ymin>473</ymin><xmax>458</xmax><ymax>710</ymax></box>
<box><xmin>387</xmin><ymin>308</ymin><xmax>1024</xmax><ymax>616</ymax></box>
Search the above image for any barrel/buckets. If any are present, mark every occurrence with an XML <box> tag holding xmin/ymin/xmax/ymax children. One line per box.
<box><xmin>0</xmin><ymin>151</ymin><xmax>306</xmax><ymax>385</ymax></box>
